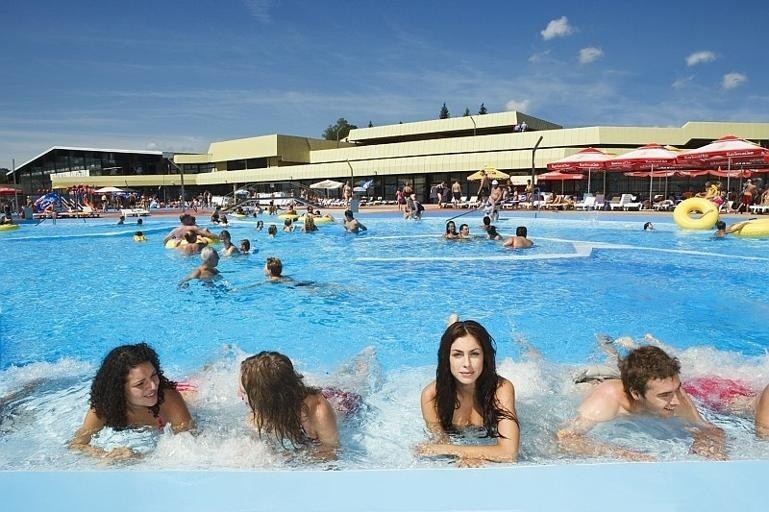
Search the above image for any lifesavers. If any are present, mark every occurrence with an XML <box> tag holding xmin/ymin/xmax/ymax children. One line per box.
<box><xmin>231</xmin><ymin>214</ymin><xmax>250</xmax><ymax>219</ymax></box>
<box><xmin>673</xmin><ymin>197</ymin><xmax>718</xmax><ymax>229</ymax></box>
<box><xmin>298</xmin><ymin>217</ymin><xmax>335</xmax><ymax>223</ymax></box>
<box><xmin>165</xmin><ymin>235</ymin><xmax>220</xmax><ymax>248</ymax></box>
<box><xmin>0</xmin><ymin>224</ymin><xmax>19</xmax><ymax>232</ymax></box>
<box><xmin>731</xmin><ymin>218</ymin><xmax>769</xmax><ymax>236</ymax></box>
<box><xmin>280</xmin><ymin>213</ymin><xmax>300</xmax><ymax>219</ymax></box>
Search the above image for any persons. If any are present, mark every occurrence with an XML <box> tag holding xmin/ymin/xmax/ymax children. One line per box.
<box><xmin>714</xmin><ymin>221</ymin><xmax>752</xmax><ymax>237</ymax></box>
<box><xmin>613</xmin><ymin>334</ymin><xmax>769</xmax><ymax>440</ymax></box>
<box><xmin>633</xmin><ymin>179</ymin><xmax>769</xmax><ymax>214</ymax></box>
<box><xmin>513</xmin><ymin>122</ymin><xmax>528</xmax><ymax>132</ymax></box>
<box><xmin>237</xmin><ymin>347</ymin><xmax>382</xmax><ymax>462</ymax></box>
<box><xmin>520</xmin><ymin>332</ymin><xmax>730</xmax><ymax>462</ymax></box>
<box><xmin>415</xmin><ymin>313</ymin><xmax>519</xmax><ymax>467</ymax></box>
<box><xmin>176</xmin><ymin>247</ymin><xmax>221</xmax><ymax>289</ymax></box>
<box><xmin>0</xmin><ymin>179</ymin><xmax>577</xmax><ymax>257</ymax></box>
<box><xmin>232</xmin><ymin>257</ymin><xmax>361</xmax><ymax>296</ymax></box>
<box><xmin>644</xmin><ymin>222</ymin><xmax>655</xmax><ymax>230</ymax></box>
<box><xmin>69</xmin><ymin>342</ymin><xmax>229</xmax><ymax>466</ymax></box>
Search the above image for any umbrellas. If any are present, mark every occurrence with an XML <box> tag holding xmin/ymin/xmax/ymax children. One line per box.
<box><xmin>538</xmin><ymin>136</ymin><xmax>769</xmax><ymax>203</ymax></box>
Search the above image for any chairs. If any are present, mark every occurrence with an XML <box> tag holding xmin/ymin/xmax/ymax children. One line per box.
<box><xmin>441</xmin><ymin>194</ymin><xmax>489</xmax><ymax>209</ymax></box>
<box><xmin>160</xmin><ymin>199</ymin><xmax>200</xmax><ymax>208</ymax></box>
<box><xmin>120</xmin><ymin>208</ymin><xmax>150</xmax><ymax>217</ymax></box>
<box><xmin>718</xmin><ymin>189</ymin><xmax>769</xmax><ymax>215</ymax></box>
<box><xmin>314</xmin><ymin>197</ymin><xmax>352</xmax><ymax>206</ymax></box>
<box><xmin>33</xmin><ymin>207</ymin><xmax>101</xmax><ymax>218</ymax></box>
<box><xmin>502</xmin><ymin>192</ymin><xmax>684</xmax><ymax>212</ymax></box>
<box><xmin>358</xmin><ymin>195</ymin><xmax>397</xmax><ymax>207</ymax></box>
